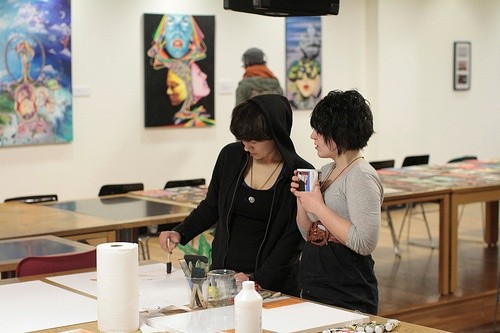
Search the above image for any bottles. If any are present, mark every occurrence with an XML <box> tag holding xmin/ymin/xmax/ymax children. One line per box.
<box><xmin>234</xmin><ymin>281</ymin><xmax>263</xmax><ymax>333</ymax></box>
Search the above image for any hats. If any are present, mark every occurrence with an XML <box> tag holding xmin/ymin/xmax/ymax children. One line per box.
<box><xmin>243</xmin><ymin>48</ymin><xmax>263</xmax><ymax>65</ymax></box>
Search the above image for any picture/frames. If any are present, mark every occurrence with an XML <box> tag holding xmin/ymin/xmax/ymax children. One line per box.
<box><xmin>454</xmin><ymin>41</ymin><xmax>471</xmax><ymax>91</ymax></box>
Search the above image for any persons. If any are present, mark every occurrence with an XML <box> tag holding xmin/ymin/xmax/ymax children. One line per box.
<box><xmin>290</xmin><ymin>89</ymin><xmax>384</xmax><ymax>316</ymax></box>
<box><xmin>236</xmin><ymin>47</ymin><xmax>284</xmax><ymax>107</ymax></box>
<box><xmin>158</xmin><ymin>94</ymin><xmax>316</xmax><ymax>299</ymax></box>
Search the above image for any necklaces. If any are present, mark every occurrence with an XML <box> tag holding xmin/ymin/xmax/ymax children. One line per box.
<box><xmin>248</xmin><ymin>155</ymin><xmax>283</xmax><ymax>203</ymax></box>
<box><xmin>319</xmin><ymin>156</ymin><xmax>364</xmax><ymax>197</ymax></box>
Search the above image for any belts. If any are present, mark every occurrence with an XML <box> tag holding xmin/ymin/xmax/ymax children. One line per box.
<box><xmin>307</xmin><ymin>220</ymin><xmax>340</xmax><ymax>246</ymax></box>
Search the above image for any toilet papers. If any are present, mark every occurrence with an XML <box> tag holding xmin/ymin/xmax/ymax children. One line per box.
<box><xmin>96</xmin><ymin>243</ymin><xmax>140</xmax><ymax>332</ymax></box>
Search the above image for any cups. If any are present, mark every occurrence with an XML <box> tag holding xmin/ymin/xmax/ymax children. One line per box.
<box><xmin>297</xmin><ymin>169</ymin><xmax>318</xmax><ymax>192</ymax></box>
<box><xmin>188</xmin><ymin>276</ymin><xmax>209</xmax><ymax>309</ymax></box>
<box><xmin>207</xmin><ymin>269</ymin><xmax>235</xmax><ymax>307</ymax></box>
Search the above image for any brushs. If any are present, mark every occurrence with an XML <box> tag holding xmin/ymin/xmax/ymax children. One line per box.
<box><xmin>188</xmin><ymin>260</ymin><xmax>207</xmax><ymax>309</ymax></box>
<box><xmin>166</xmin><ymin>239</ymin><xmax>172</xmax><ymax>273</ymax></box>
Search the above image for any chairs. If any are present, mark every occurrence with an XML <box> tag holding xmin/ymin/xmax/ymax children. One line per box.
<box><xmin>4</xmin><ymin>155</ymin><xmax>479</xmax><ymax>275</ymax></box>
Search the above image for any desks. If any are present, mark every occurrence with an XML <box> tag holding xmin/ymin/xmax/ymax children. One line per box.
<box><xmin>1</xmin><ymin>259</ymin><xmax>455</xmax><ymax>333</ymax></box>
<box><xmin>0</xmin><ymin>160</ymin><xmax>499</xmax><ymax>294</ymax></box>
